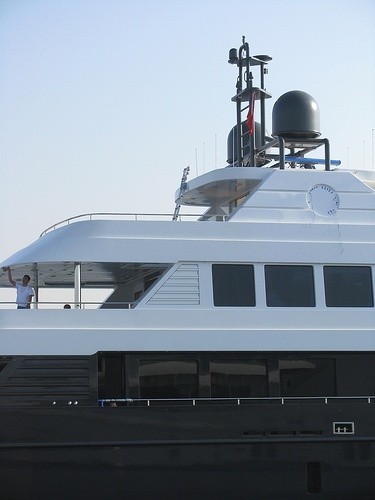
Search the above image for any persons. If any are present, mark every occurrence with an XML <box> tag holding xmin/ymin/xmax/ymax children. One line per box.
<box><xmin>6</xmin><ymin>267</ymin><xmax>34</xmax><ymax>309</ymax></box>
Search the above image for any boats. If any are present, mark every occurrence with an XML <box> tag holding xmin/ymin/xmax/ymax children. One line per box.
<box><xmin>0</xmin><ymin>35</ymin><xmax>375</xmax><ymax>499</ymax></box>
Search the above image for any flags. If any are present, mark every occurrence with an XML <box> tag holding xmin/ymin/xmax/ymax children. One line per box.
<box><xmin>245</xmin><ymin>91</ymin><xmax>255</xmax><ymax>136</ymax></box>
